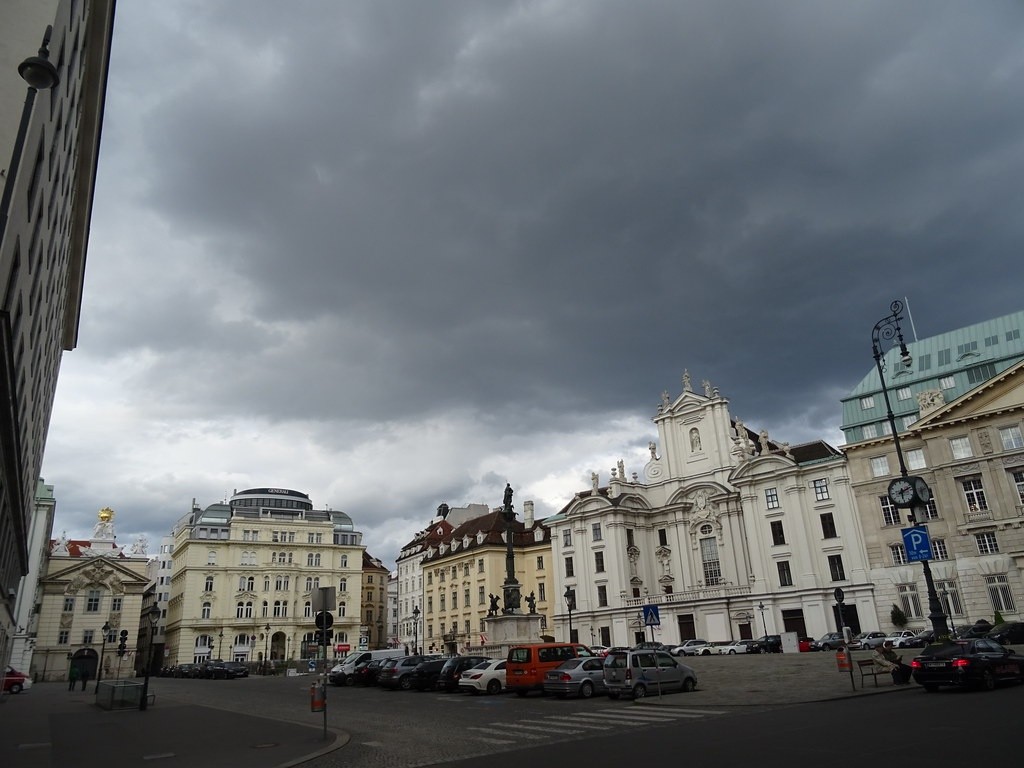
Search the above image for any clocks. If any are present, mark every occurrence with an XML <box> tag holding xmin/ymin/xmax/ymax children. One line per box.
<box><xmin>915</xmin><ymin>477</ymin><xmax>931</xmax><ymax>506</ymax></box>
<box><xmin>887</xmin><ymin>478</ymin><xmax>915</xmax><ymax>508</ymax></box>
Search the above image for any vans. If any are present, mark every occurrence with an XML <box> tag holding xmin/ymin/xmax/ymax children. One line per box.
<box><xmin>329</xmin><ymin>649</ymin><xmax>406</xmax><ymax>688</ymax></box>
<box><xmin>505</xmin><ymin>642</ymin><xmax>597</xmax><ymax>694</ymax></box>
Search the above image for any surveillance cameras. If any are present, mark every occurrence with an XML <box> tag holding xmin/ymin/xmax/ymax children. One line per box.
<box><xmin>8</xmin><ymin>588</ymin><xmax>16</xmax><ymax>597</ymax></box>
<box><xmin>19</xmin><ymin>625</ymin><xmax>25</xmax><ymax>630</ymax></box>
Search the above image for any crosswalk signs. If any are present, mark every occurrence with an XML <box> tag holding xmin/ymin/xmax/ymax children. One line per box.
<box><xmin>642</xmin><ymin>604</ymin><xmax>661</xmax><ymax>625</ymax></box>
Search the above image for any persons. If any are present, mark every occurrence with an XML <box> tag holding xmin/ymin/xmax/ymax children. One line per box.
<box><xmin>489</xmin><ymin>593</ymin><xmax>499</xmax><ymax>615</ymax></box>
<box><xmin>735</xmin><ymin>417</ymin><xmax>748</xmax><ymax>438</ymax></box>
<box><xmin>648</xmin><ymin>441</ymin><xmax>656</xmax><ymax>458</ymax></box>
<box><xmin>503</xmin><ymin>483</ymin><xmax>514</xmax><ymax>512</ymax></box>
<box><xmin>692</xmin><ymin>430</ymin><xmax>701</xmax><ymax>452</ymax></box>
<box><xmin>527</xmin><ymin>592</ymin><xmax>536</xmax><ymax>613</ymax></box>
<box><xmin>758</xmin><ymin>429</ymin><xmax>770</xmax><ymax>455</ymax></box>
<box><xmin>592</xmin><ymin>473</ymin><xmax>600</xmax><ymax>495</ymax></box>
<box><xmin>67</xmin><ymin>663</ymin><xmax>90</xmax><ymax>691</ymax></box>
<box><xmin>617</xmin><ymin>460</ymin><xmax>624</xmax><ymax>478</ymax></box>
<box><xmin>702</xmin><ymin>378</ymin><xmax>711</xmax><ymax>398</ymax></box>
<box><xmin>269</xmin><ymin>656</ymin><xmax>276</xmax><ymax>676</ymax></box>
<box><xmin>682</xmin><ymin>368</ymin><xmax>692</xmax><ymax>391</ymax></box>
<box><xmin>916</xmin><ymin>389</ymin><xmax>945</xmax><ymax>410</ymax></box>
<box><xmin>660</xmin><ymin>388</ymin><xmax>670</xmax><ymax>405</ymax></box>
<box><xmin>405</xmin><ymin>646</ymin><xmax>419</xmax><ymax>656</ymax></box>
<box><xmin>872</xmin><ymin>640</ymin><xmax>912</xmax><ymax>685</ymax></box>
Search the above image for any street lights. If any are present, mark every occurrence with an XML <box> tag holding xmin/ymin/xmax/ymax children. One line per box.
<box><xmin>413</xmin><ymin>606</ymin><xmax>421</xmax><ymax>656</ymax></box>
<box><xmin>42</xmin><ymin>649</ymin><xmax>50</xmax><ymax>682</ymax></box>
<box><xmin>141</xmin><ymin>601</ymin><xmax>161</xmax><ymax>709</ymax></box>
<box><xmin>96</xmin><ymin>620</ymin><xmax>111</xmax><ymax>694</ymax></box>
<box><xmin>564</xmin><ymin>586</ymin><xmax>574</xmax><ymax>641</ymax></box>
<box><xmin>870</xmin><ymin>299</ymin><xmax>952</xmax><ymax>639</ymax></box>
<box><xmin>264</xmin><ymin>622</ymin><xmax>270</xmax><ymax>666</ymax></box>
<box><xmin>219</xmin><ymin>631</ymin><xmax>224</xmax><ymax>658</ymax></box>
<box><xmin>758</xmin><ymin>601</ymin><xmax>769</xmax><ymax>640</ymax></box>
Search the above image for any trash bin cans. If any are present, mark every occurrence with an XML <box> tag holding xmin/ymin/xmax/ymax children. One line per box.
<box><xmin>835</xmin><ymin>646</ymin><xmax>851</xmax><ymax>672</ymax></box>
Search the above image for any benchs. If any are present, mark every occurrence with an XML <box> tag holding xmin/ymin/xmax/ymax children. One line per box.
<box><xmin>857</xmin><ymin>659</ymin><xmax>891</xmax><ymax>687</ymax></box>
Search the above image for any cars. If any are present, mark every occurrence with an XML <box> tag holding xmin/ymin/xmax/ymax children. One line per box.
<box><xmin>353</xmin><ymin>654</ymin><xmax>524</xmax><ymax>695</ymax></box>
<box><xmin>577</xmin><ymin>633</ymin><xmax>815</xmax><ymax>654</ymax></box>
<box><xmin>162</xmin><ymin>660</ymin><xmax>279</xmax><ymax>678</ymax></box>
<box><xmin>809</xmin><ymin>621</ymin><xmax>1023</xmax><ymax>651</ymax></box>
<box><xmin>542</xmin><ymin>649</ymin><xmax>695</xmax><ymax>699</ymax></box>
<box><xmin>911</xmin><ymin>638</ymin><xmax>1024</xmax><ymax>692</ymax></box>
<box><xmin>1</xmin><ymin>665</ymin><xmax>30</xmax><ymax>694</ymax></box>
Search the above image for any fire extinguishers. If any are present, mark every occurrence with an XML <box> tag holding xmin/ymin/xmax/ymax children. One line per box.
<box><xmin>309</xmin><ymin>677</ymin><xmax>324</xmax><ymax>714</ymax></box>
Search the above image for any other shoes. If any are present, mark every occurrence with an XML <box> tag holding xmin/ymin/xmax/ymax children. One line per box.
<box><xmin>894</xmin><ymin>682</ymin><xmax>906</xmax><ymax>686</ymax></box>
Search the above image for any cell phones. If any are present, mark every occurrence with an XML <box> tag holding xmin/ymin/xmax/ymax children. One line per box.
<box><xmin>898</xmin><ymin>654</ymin><xmax>904</xmax><ymax>660</ymax></box>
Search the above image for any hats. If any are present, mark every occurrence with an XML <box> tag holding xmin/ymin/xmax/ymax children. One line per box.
<box><xmin>885</xmin><ymin>641</ymin><xmax>896</xmax><ymax>646</ymax></box>
